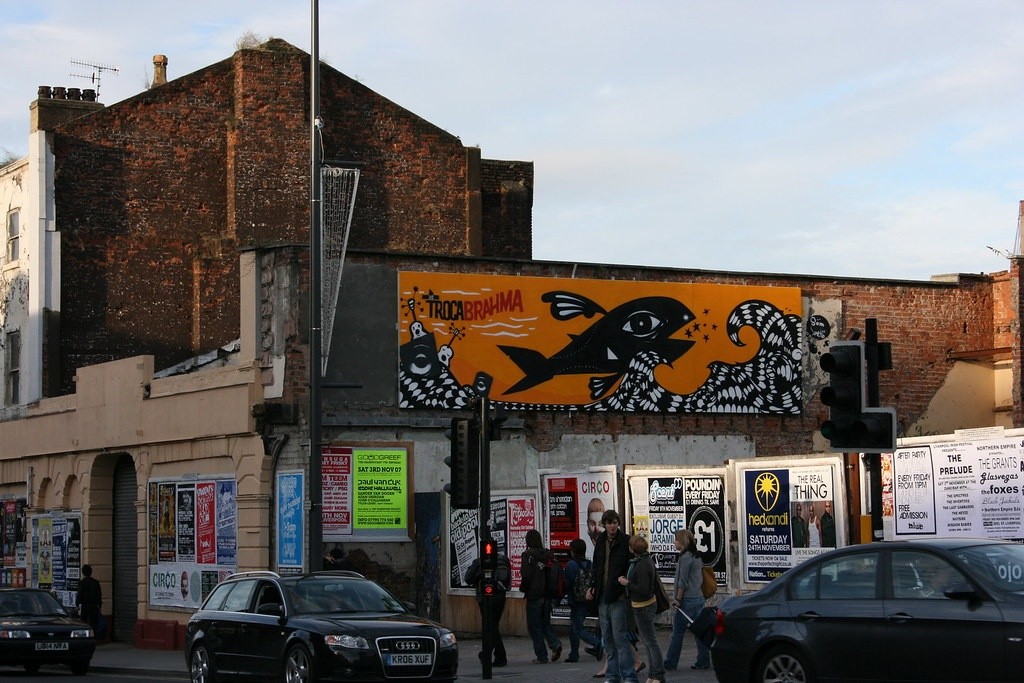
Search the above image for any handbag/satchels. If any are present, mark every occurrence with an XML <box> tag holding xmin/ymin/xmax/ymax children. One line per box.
<box><xmin>653</xmin><ymin>574</ymin><xmax>670</xmax><ymax>615</ymax></box>
<box><xmin>699</xmin><ymin>566</ymin><xmax>718</xmax><ymax>598</ymax></box>
<box><xmin>496</xmin><ymin>554</ymin><xmax>511</xmax><ymax>593</ymax></box>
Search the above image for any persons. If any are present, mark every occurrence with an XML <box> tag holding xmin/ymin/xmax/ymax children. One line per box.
<box><xmin>820</xmin><ymin>502</ymin><xmax>836</xmax><ymax>548</ymax></box>
<box><xmin>791</xmin><ymin>504</ymin><xmax>807</xmax><ymax>548</ymax></box>
<box><xmin>617</xmin><ymin>535</ymin><xmax>666</xmax><ymax>683</ymax></box>
<box><xmin>585</xmin><ymin>510</ymin><xmax>638</xmax><ymax>683</ymax></box>
<box><xmin>180</xmin><ymin>571</ymin><xmax>189</xmax><ymax>601</ymax></box>
<box><xmin>806</xmin><ymin>504</ymin><xmax>822</xmax><ymax>548</ymax></box>
<box><xmin>563</xmin><ymin>537</ymin><xmax>603</xmax><ymax>665</ymax></box>
<box><xmin>664</xmin><ymin>530</ymin><xmax>711</xmax><ymax>671</ymax></box>
<box><xmin>518</xmin><ymin>530</ymin><xmax>562</xmax><ymax>664</ymax></box>
<box><xmin>464</xmin><ymin>536</ymin><xmax>512</xmax><ymax>667</ymax></box>
<box><xmin>75</xmin><ymin>564</ymin><xmax>103</xmax><ymax>627</ymax></box>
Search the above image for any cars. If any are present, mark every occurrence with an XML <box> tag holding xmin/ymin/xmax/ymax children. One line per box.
<box><xmin>0</xmin><ymin>588</ymin><xmax>95</xmax><ymax>677</ymax></box>
<box><xmin>712</xmin><ymin>538</ymin><xmax>1024</xmax><ymax>683</ymax></box>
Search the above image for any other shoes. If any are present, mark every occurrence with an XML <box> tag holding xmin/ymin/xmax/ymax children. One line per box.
<box><xmin>645</xmin><ymin>677</ymin><xmax>665</xmax><ymax>683</ymax></box>
<box><xmin>565</xmin><ymin>658</ymin><xmax>578</xmax><ymax>663</ymax></box>
<box><xmin>492</xmin><ymin>662</ymin><xmax>507</xmax><ymax>667</ymax></box>
<box><xmin>595</xmin><ymin>641</ymin><xmax>603</xmax><ymax>661</ymax></box>
<box><xmin>593</xmin><ymin>673</ymin><xmax>605</xmax><ymax>677</ymax></box>
<box><xmin>634</xmin><ymin>662</ymin><xmax>645</xmax><ymax>673</ymax></box>
<box><xmin>531</xmin><ymin>659</ymin><xmax>548</xmax><ymax>664</ymax></box>
<box><xmin>551</xmin><ymin>646</ymin><xmax>562</xmax><ymax>661</ymax></box>
<box><xmin>691</xmin><ymin>666</ymin><xmax>711</xmax><ymax>670</ymax></box>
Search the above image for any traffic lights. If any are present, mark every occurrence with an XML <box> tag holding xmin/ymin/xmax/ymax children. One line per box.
<box><xmin>819</xmin><ymin>344</ymin><xmax>897</xmax><ymax>454</ymax></box>
<box><xmin>444</xmin><ymin>416</ymin><xmax>478</xmax><ymax>510</ymax></box>
<box><xmin>482</xmin><ymin>541</ymin><xmax>498</xmax><ymax>596</ymax></box>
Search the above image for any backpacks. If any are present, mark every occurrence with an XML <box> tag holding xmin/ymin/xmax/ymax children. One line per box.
<box><xmin>525</xmin><ymin>548</ymin><xmax>566</xmax><ymax>600</ymax></box>
<box><xmin>572</xmin><ymin>559</ymin><xmax>595</xmax><ymax>602</ymax></box>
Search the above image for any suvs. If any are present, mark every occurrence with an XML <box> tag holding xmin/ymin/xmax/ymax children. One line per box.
<box><xmin>183</xmin><ymin>570</ymin><xmax>458</xmax><ymax>683</ymax></box>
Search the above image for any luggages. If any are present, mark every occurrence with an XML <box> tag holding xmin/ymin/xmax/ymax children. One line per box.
<box><xmin>672</xmin><ymin>603</ymin><xmax>720</xmax><ymax>649</ymax></box>
